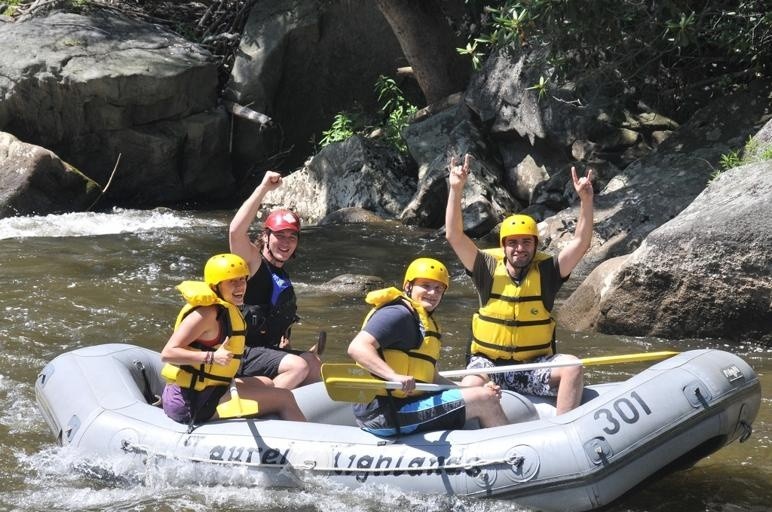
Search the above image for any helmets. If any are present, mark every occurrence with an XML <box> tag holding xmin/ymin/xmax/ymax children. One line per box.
<box><xmin>500</xmin><ymin>215</ymin><xmax>540</xmax><ymax>248</ymax></box>
<box><xmin>204</xmin><ymin>253</ymin><xmax>250</xmax><ymax>287</ymax></box>
<box><xmin>264</xmin><ymin>209</ymin><xmax>300</xmax><ymax>232</ymax></box>
<box><xmin>402</xmin><ymin>258</ymin><xmax>449</xmax><ymax>294</ymax></box>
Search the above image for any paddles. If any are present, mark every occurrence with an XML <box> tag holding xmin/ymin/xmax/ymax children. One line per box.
<box><xmin>438</xmin><ymin>351</ymin><xmax>684</xmax><ymax>378</ymax></box>
<box><xmin>320</xmin><ymin>362</ymin><xmax>484</xmax><ymax>404</ymax></box>
<box><xmin>216</xmin><ymin>376</ymin><xmax>260</xmax><ymax>420</ymax></box>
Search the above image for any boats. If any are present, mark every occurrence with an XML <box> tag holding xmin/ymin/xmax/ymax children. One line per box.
<box><xmin>32</xmin><ymin>341</ymin><xmax>763</xmax><ymax>512</ymax></box>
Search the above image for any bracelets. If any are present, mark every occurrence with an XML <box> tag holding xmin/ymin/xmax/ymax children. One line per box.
<box><xmin>210</xmin><ymin>352</ymin><xmax>215</xmax><ymax>364</ymax></box>
<box><xmin>204</xmin><ymin>351</ymin><xmax>210</xmax><ymax>363</ymax></box>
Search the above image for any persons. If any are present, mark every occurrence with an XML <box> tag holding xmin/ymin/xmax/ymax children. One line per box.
<box><xmin>228</xmin><ymin>170</ymin><xmax>323</xmax><ymax>390</ymax></box>
<box><xmin>346</xmin><ymin>257</ymin><xmax>511</xmax><ymax>436</ymax></box>
<box><xmin>444</xmin><ymin>154</ymin><xmax>595</xmax><ymax>414</ymax></box>
<box><xmin>160</xmin><ymin>253</ymin><xmax>307</xmax><ymax>423</ymax></box>
